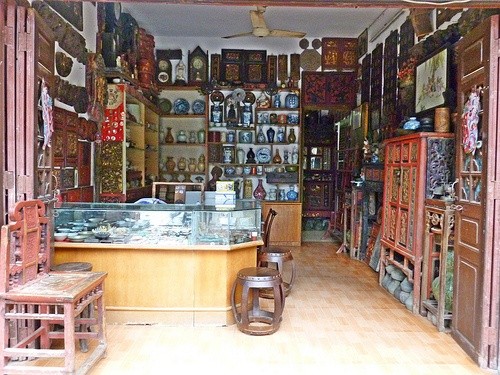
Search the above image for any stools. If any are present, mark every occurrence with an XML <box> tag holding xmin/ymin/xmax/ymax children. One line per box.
<box><xmin>258</xmin><ymin>245</ymin><xmax>294</xmax><ymax>299</ymax></box>
<box><xmin>231</xmin><ymin>266</ymin><xmax>284</xmax><ymax>336</ymax></box>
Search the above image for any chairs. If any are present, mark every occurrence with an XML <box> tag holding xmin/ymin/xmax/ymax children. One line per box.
<box><xmin>260</xmin><ymin>208</ymin><xmax>278</xmax><ymax>249</ymax></box>
<box><xmin>152</xmin><ymin>181</ymin><xmax>205</xmax><ymax>205</ymax></box>
<box><xmin>0</xmin><ymin>198</ymin><xmax>106</xmax><ymax>375</ymax></box>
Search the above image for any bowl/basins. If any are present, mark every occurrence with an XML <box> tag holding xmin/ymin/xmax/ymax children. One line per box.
<box><xmin>53</xmin><ymin>227</ymin><xmax>94</xmax><ymax>242</ymax></box>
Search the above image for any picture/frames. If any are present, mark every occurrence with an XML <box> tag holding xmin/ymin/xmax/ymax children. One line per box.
<box><xmin>413</xmin><ymin>43</ymin><xmax>452</xmax><ymax>116</ymax></box>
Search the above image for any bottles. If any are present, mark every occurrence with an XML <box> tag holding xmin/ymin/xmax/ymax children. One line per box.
<box><xmin>158</xmin><ymin>153</ymin><xmax>205</xmax><ymax>172</ymax></box>
<box><xmin>403</xmin><ymin>117</ymin><xmax>432</xmax><ymax>132</ymax></box>
<box><xmin>160</xmin><ymin>126</ymin><xmax>206</xmax><ymax>144</ymax></box>
<box><xmin>206</xmin><ymin>93</ymin><xmax>299</xmax><ymax>202</ymax></box>
<box><xmin>159</xmin><ymin>173</ymin><xmax>204</xmax><ymax>192</ymax></box>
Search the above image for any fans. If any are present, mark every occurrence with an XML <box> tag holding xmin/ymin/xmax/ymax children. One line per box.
<box><xmin>222</xmin><ymin>4</ymin><xmax>306</xmax><ymax>40</ymax></box>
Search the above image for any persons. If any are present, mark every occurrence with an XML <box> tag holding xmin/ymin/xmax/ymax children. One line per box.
<box><xmin>176</xmin><ymin>60</ymin><xmax>186</xmax><ymax>80</ymax></box>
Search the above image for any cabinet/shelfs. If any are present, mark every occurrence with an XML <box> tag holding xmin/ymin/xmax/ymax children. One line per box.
<box><xmin>100</xmin><ymin>83</ymin><xmax>457</xmax><ymax>331</ymax></box>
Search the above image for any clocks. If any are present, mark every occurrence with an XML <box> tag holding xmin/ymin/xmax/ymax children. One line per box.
<box><xmin>154</xmin><ymin>58</ymin><xmax>173</xmax><ymax>86</ymax></box>
<box><xmin>186</xmin><ymin>45</ymin><xmax>209</xmax><ymax>86</ymax></box>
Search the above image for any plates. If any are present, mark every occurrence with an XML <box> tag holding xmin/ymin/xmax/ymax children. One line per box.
<box><xmin>158</xmin><ymin>97</ymin><xmax>205</xmax><ymax>114</ymax></box>
<box><xmin>256</xmin><ymin>147</ymin><xmax>270</xmax><ymax>163</ymax></box>
